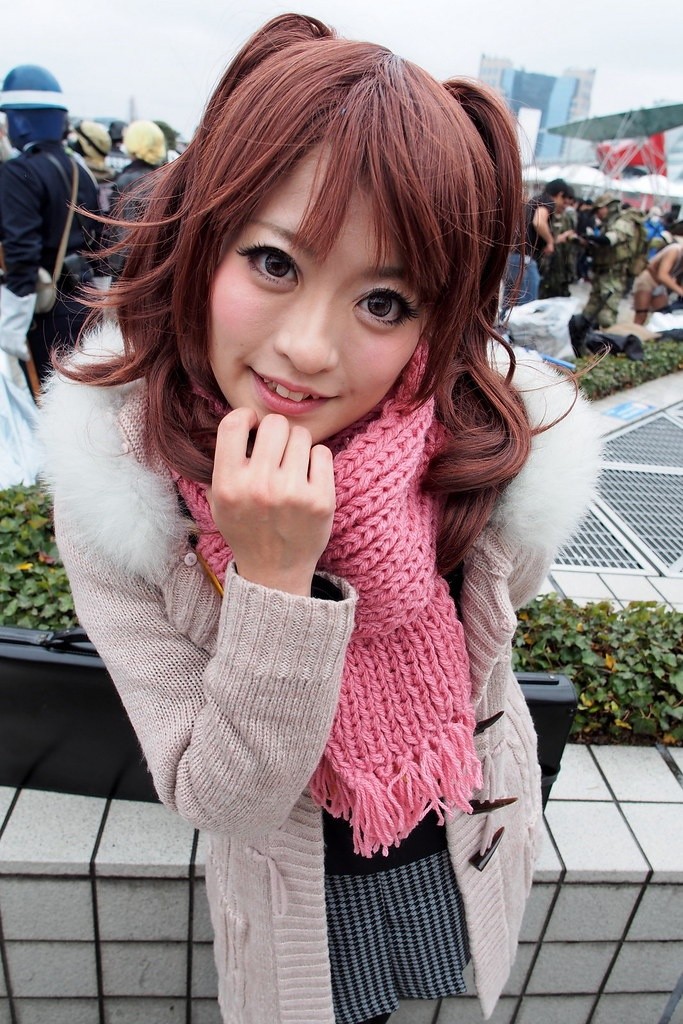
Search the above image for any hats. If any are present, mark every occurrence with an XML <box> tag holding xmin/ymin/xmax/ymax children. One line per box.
<box><xmin>106</xmin><ymin>119</ymin><xmax>129</xmax><ymax>140</ymax></box>
<box><xmin>68</xmin><ymin>118</ymin><xmax>112</xmax><ymax>162</ymax></box>
<box><xmin>122</xmin><ymin>120</ymin><xmax>168</xmax><ymax>166</ymax></box>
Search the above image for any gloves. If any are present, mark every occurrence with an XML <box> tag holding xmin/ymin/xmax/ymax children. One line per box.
<box><xmin>0</xmin><ymin>284</ymin><xmax>37</xmax><ymax>362</ymax></box>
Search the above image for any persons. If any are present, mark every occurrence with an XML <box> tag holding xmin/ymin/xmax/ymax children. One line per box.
<box><xmin>500</xmin><ymin>178</ymin><xmax>683</xmax><ymax>356</ymax></box>
<box><xmin>37</xmin><ymin>13</ymin><xmax>602</xmax><ymax>1024</ymax></box>
<box><xmin>0</xmin><ymin>65</ymin><xmax>205</xmax><ymax>492</ymax></box>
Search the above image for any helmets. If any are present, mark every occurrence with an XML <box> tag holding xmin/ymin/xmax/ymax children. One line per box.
<box><xmin>0</xmin><ymin>62</ymin><xmax>69</xmax><ymax>110</ymax></box>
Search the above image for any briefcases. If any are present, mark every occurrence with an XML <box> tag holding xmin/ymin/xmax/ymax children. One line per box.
<box><xmin>513</xmin><ymin>671</ymin><xmax>578</xmax><ymax>817</ymax></box>
<box><xmin>0</xmin><ymin>624</ymin><xmax>167</xmax><ymax>803</ymax></box>
